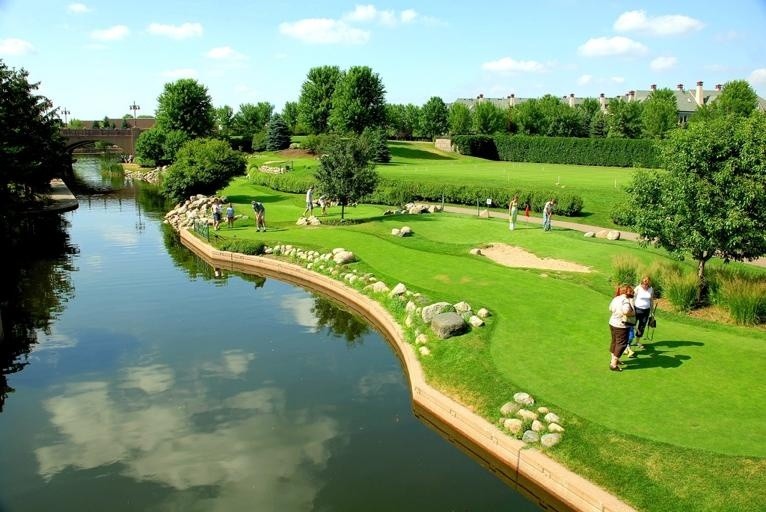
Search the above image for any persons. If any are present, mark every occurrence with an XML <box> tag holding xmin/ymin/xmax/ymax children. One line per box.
<box><xmin>211</xmin><ymin>198</ymin><xmax>234</xmax><ymax>231</ymax></box>
<box><xmin>303</xmin><ymin>186</ymin><xmax>331</xmax><ymax>217</ymax></box>
<box><xmin>609</xmin><ymin>275</ymin><xmax>654</xmax><ymax>372</ymax></box>
<box><xmin>543</xmin><ymin>199</ymin><xmax>557</xmax><ymax>230</ymax></box>
<box><xmin>509</xmin><ymin>196</ymin><xmax>519</xmax><ymax>230</ymax></box>
<box><xmin>252</xmin><ymin>201</ymin><xmax>266</xmax><ymax>232</ymax></box>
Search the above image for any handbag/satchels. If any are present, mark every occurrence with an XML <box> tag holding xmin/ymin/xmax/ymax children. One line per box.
<box><xmin>648</xmin><ymin>316</ymin><xmax>656</xmax><ymax>327</ymax></box>
<box><xmin>621</xmin><ymin>317</ymin><xmax>636</xmax><ymax>326</ymax></box>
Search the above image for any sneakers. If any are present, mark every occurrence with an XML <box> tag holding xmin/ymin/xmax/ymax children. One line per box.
<box><xmin>256</xmin><ymin>229</ymin><xmax>266</xmax><ymax>232</ymax></box>
<box><xmin>624</xmin><ymin>348</ymin><xmax>635</xmax><ymax>357</ymax></box>
<box><xmin>609</xmin><ymin>359</ymin><xmax>624</xmax><ymax>371</ymax></box>
